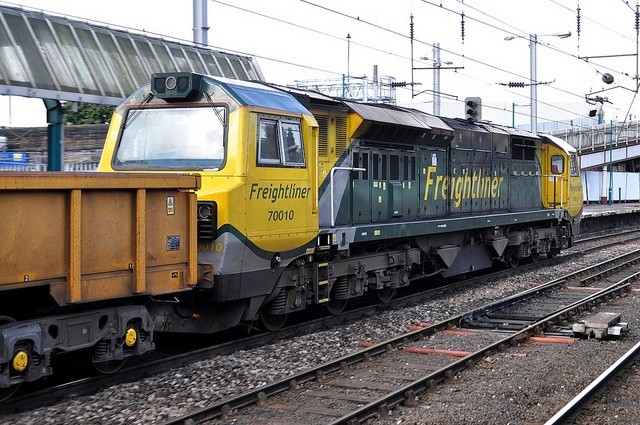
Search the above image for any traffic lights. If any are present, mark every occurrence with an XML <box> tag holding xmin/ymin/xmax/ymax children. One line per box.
<box><xmin>463</xmin><ymin>97</ymin><xmax>482</xmax><ymax>121</ymax></box>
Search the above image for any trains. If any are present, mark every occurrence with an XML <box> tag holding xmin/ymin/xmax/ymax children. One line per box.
<box><xmin>1</xmin><ymin>69</ymin><xmax>585</xmax><ymax>392</ymax></box>
